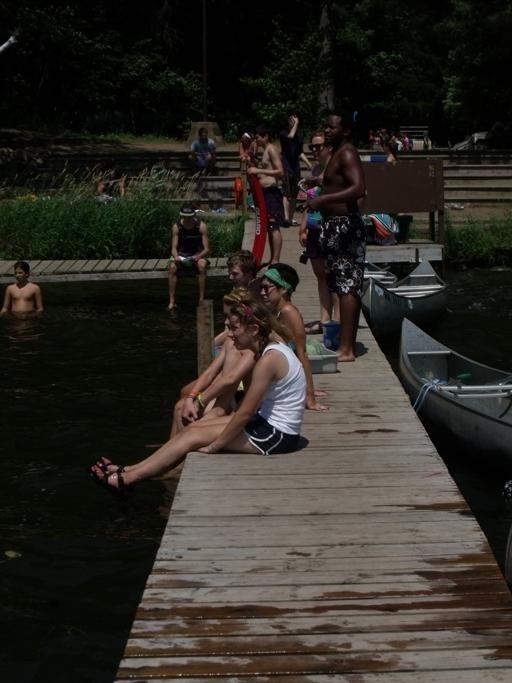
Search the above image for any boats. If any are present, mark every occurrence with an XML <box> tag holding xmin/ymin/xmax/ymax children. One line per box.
<box><xmin>392</xmin><ymin>315</ymin><xmax>510</xmax><ymax>459</ymax></box>
<box><xmin>356</xmin><ymin>255</ymin><xmax>451</xmax><ymax>327</ymax></box>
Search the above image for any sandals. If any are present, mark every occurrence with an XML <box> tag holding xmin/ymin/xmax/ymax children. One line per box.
<box><xmin>94</xmin><ymin>455</ymin><xmax>128</xmax><ymax>474</ymax></box>
<box><xmin>89</xmin><ymin>462</ymin><xmax>131</xmax><ymax>498</ymax></box>
<box><xmin>304</xmin><ymin>319</ymin><xmax>328</xmax><ymax>335</ymax></box>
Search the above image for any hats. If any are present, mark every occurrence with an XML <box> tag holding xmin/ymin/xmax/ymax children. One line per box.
<box><xmin>178</xmin><ymin>204</ymin><xmax>196</xmax><ymax>217</ymax></box>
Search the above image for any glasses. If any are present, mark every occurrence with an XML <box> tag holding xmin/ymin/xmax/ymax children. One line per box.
<box><xmin>259</xmin><ymin>282</ymin><xmax>276</xmax><ymax>293</ymax></box>
<box><xmin>308</xmin><ymin>143</ymin><xmax>327</xmax><ymax>151</ymax></box>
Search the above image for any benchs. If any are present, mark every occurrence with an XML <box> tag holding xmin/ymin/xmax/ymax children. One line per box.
<box><xmin>399</xmin><ymin>125</ymin><xmax>430</xmax><ymax>143</ymax></box>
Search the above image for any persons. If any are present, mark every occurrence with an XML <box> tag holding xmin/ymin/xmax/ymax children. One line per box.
<box><xmin>0</xmin><ymin>260</ymin><xmax>44</xmax><ymax>315</ymax></box>
